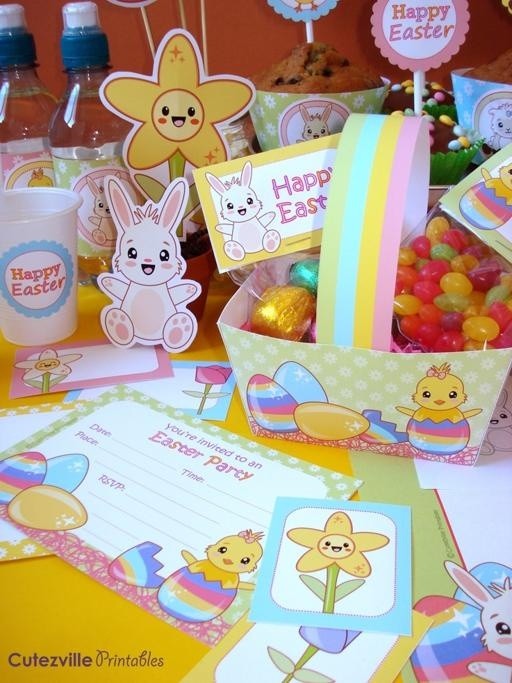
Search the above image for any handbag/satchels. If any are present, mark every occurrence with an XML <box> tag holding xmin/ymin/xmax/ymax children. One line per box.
<box><xmin>217</xmin><ymin>113</ymin><xmax>512</xmax><ymax>464</ymax></box>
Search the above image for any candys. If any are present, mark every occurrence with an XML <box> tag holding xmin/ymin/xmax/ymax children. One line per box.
<box><xmin>245</xmin><ymin>215</ymin><xmax>512</xmax><ymax>352</ymax></box>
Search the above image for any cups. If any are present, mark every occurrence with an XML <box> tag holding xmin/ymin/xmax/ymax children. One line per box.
<box><xmin>0</xmin><ymin>187</ymin><xmax>89</xmax><ymax>346</ymax></box>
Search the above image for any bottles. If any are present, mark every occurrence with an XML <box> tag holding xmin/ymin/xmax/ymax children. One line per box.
<box><xmin>0</xmin><ymin>4</ymin><xmax>60</xmax><ymax>187</ymax></box>
<box><xmin>49</xmin><ymin>0</ymin><xmax>146</xmax><ymax>286</ymax></box>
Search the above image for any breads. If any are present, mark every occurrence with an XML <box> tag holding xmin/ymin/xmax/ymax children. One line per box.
<box><xmin>247</xmin><ymin>43</ymin><xmax>385</xmax><ymax>92</ymax></box>
<box><xmin>381</xmin><ymin>78</ymin><xmax>473</xmax><ymax>154</ymax></box>
